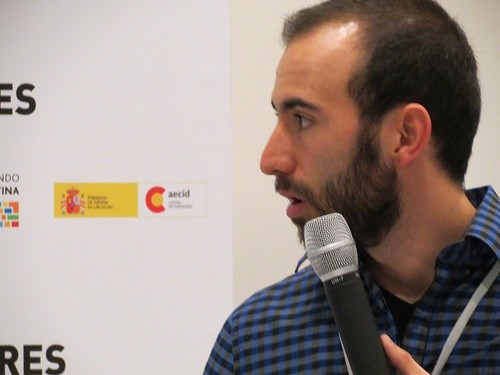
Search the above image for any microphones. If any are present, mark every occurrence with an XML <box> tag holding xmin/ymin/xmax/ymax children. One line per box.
<box><xmin>302</xmin><ymin>212</ymin><xmax>395</xmax><ymax>374</ymax></box>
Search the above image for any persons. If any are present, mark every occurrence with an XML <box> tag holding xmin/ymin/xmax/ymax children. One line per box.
<box><xmin>200</xmin><ymin>0</ymin><xmax>500</xmax><ymax>375</ymax></box>
